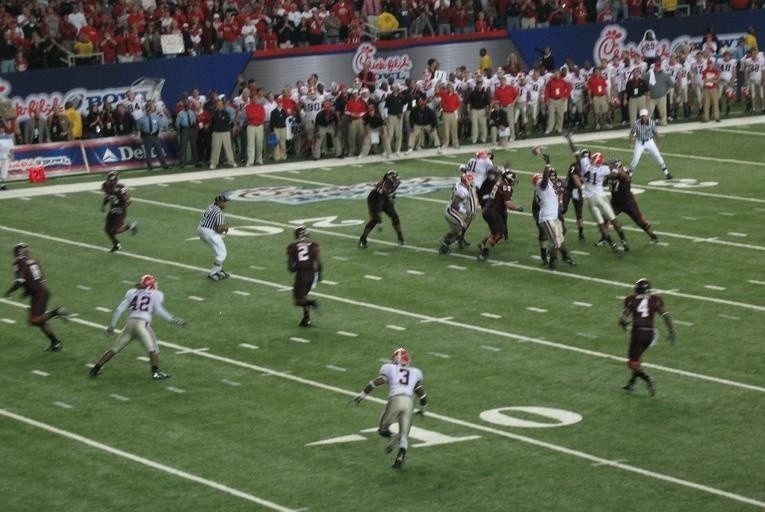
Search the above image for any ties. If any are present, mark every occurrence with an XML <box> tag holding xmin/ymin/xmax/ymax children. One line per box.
<box><xmin>187</xmin><ymin>111</ymin><xmax>191</xmax><ymax>126</ymax></box>
<box><xmin>148</xmin><ymin>115</ymin><xmax>152</xmax><ymax>132</ymax></box>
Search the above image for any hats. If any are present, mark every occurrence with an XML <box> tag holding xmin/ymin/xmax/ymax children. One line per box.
<box><xmin>215</xmin><ymin>194</ymin><xmax>230</xmax><ymax>202</ymax></box>
<box><xmin>639</xmin><ymin>109</ymin><xmax>648</xmax><ymax>116</ymax></box>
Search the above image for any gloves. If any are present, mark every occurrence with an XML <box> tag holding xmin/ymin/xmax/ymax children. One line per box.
<box><xmin>104</xmin><ymin>328</ymin><xmax>115</xmax><ymax>337</ymax></box>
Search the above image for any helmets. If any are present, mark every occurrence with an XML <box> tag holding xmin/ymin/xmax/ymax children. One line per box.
<box><xmin>391</xmin><ymin>348</ymin><xmax>410</xmax><ymax>366</ymax></box>
<box><xmin>108</xmin><ymin>170</ymin><xmax>120</xmax><ymax>181</ymax></box>
<box><xmin>532</xmin><ymin>149</ymin><xmax>622</xmax><ymax>185</ymax></box>
<box><xmin>296</xmin><ymin>226</ymin><xmax>309</xmax><ymax>238</ymax></box>
<box><xmin>14</xmin><ymin>242</ymin><xmax>29</xmax><ymax>258</ymax></box>
<box><xmin>461</xmin><ymin>150</ymin><xmax>517</xmax><ymax>187</ymax></box>
<box><xmin>635</xmin><ymin>278</ymin><xmax>651</xmax><ymax>293</ymax></box>
<box><xmin>388</xmin><ymin>170</ymin><xmax>400</xmax><ymax>183</ymax></box>
<box><xmin>141</xmin><ymin>275</ymin><xmax>154</xmax><ymax>286</ymax></box>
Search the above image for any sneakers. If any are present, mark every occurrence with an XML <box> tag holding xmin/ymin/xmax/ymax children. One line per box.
<box><xmin>47</xmin><ymin>342</ymin><xmax>63</xmax><ymax>352</ymax></box>
<box><xmin>563</xmin><ymin>256</ymin><xmax>576</xmax><ymax>266</ymax></box>
<box><xmin>622</xmin><ymin>382</ymin><xmax>636</xmax><ymax>390</ymax></box>
<box><xmin>455</xmin><ymin>239</ymin><xmax>470</xmax><ymax>245</ymax></box>
<box><xmin>442</xmin><ymin>237</ymin><xmax>451</xmax><ymax>247</ymax></box>
<box><xmin>301</xmin><ymin>318</ymin><xmax>310</xmax><ymax>326</ymax></box>
<box><xmin>392</xmin><ymin>456</ymin><xmax>404</xmax><ymax>468</ymax></box>
<box><xmin>594</xmin><ymin>234</ymin><xmax>630</xmax><ymax>252</ymax></box>
<box><xmin>110</xmin><ymin>242</ymin><xmax>121</xmax><ymax>252</ymax></box>
<box><xmin>664</xmin><ymin>172</ymin><xmax>673</xmax><ymax>179</ymax></box>
<box><xmin>359</xmin><ymin>239</ymin><xmax>367</xmax><ymax>249</ymax></box>
<box><xmin>152</xmin><ymin>371</ymin><xmax>169</xmax><ymax>380</ymax></box>
<box><xmin>208</xmin><ymin>270</ymin><xmax>229</xmax><ymax>280</ymax></box>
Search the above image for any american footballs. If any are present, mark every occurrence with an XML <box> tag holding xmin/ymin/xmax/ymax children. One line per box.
<box><xmin>533</xmin><ymin>147</ymin><xmax>541</xmax><ymax>155</ymax></box>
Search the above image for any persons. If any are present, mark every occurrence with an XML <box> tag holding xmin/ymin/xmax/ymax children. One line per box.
<box><xmin>532</xmin><ymin>132</ymin><xmax>659</xmax><ymax>270</ymax></box>
<box><xmin>2</xmin><ymin>1</ymin><xmax>763</xmax><ymax>170</ymax></box>
<box><xmin>197</xmin><ymin>195</ymin><xmax>230</xmax><ymax>280</ymax></box>
<box><xmin>360</xmin><ymin>170</ymin><xmax>404</xmax><ymax>248</ymax></box>
<box><xmin>2</xmin><ymin>242</ymin><xmax>69</xmax><ymax>352</ymax></box>
<box><xmin>287</xmin><ymin>225</ymin><xmax>323</xmax><ymax>326</ymax></box>
<box><xmin>348</xmin><ymin>347</ymin><xmax>428</xmax><ymax>468</ymax></box>
<box><xmin>89</xmin><ymin>274</ymin><xmax>187</xmax><ymax>379</ymax></box>
<box><xmin>440</xmin><ymin>150</ymin><xmax>523</xmax><ymax>259</ymax></box>
<box><xmin>629</xmin><ymin>109</ymin><xmax>674</xmax><ymax>179</ymax></box>
<box><xmin>101</xmin><ymin>171</ymin><xmax>138</xmax><ymax>252</ymax></box>
<box><xmin>618</xmin><ymin>278</ymin><xmax>677</xmax><ymax>395</ymax></box>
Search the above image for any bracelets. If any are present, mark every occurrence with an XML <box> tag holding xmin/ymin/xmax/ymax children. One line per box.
<box><xmin>419</xmin><ymin>405</ymin><xmax>427</xmax><ymax>412</ymax></box>
<box><xmin>359</xmin><ymin>390</ymin><xmax>366</xmax><ymax>399</ymax></box>
